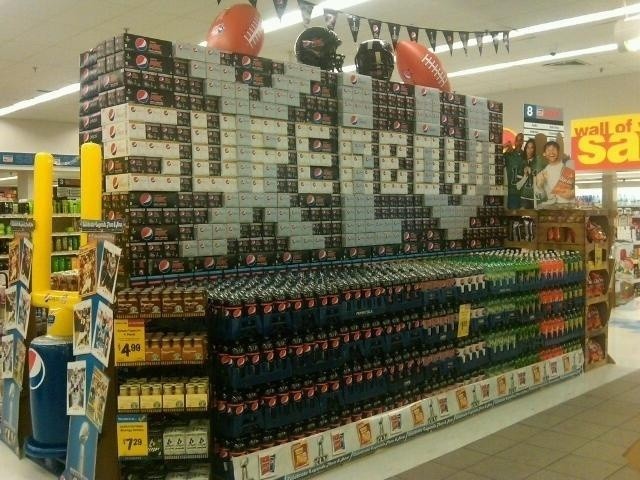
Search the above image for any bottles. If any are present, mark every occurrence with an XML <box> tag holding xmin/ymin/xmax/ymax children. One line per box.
<box><xmin>206</xmin><ymin>247</ymin><xmax>585</xmax><ymax>480</ymax></box>
<box><xmin>0</xmin><ymin>188</ymin><xmax>19</xmax><ymax>269</ymax></box>
<box><xmin>36</xmin><ymin>307</ymin><xmax>47</xmax><ymax>323</ymax></box>
<box><xmin>29</xmin><ymin>307</ymin><xmax>73</xmax><ymax>446</ymax></box>
<box><xmin>115</xmin><ymin>285</ymin><xmax>209</xmax><ymax>397</ymax></box>
<box><xmin>52</xmin><ymin>196</ymin><xmax>81</xmax><ymax>272</ymax></box>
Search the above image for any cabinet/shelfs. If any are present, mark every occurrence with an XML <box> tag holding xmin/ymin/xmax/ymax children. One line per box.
<box><xmin>540</xmin><ymin>207</ymin><xmax>619</xmax><ymax>372</ymax></box>
<box><xmin>1</xmin><ymin>197</ymin><xmax>82</xmax><ymax>276</ymax></box>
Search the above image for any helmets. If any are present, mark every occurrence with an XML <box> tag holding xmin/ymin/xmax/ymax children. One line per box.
<box><xmin>293</xmin><ymin>26</ymin><xmax>346</xmax><ymax>73</ymax></box>
<box><xmin>354</xmin><ymin>38</ymin><xmax>395</xmax><ymax>81</ymax></box>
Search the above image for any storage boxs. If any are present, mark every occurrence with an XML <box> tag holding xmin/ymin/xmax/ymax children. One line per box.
<box><xmin>67</xmin><ymin>30</ymin><xmax>504</xmax><ymax>287</ymax></box>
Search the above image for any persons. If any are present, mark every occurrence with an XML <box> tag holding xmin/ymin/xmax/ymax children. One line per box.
<box><xmin>517</xmin><ymin>137</ymin><xmax>546</xmax><ymax>209</ymax></box>
<box><xmin>531</xmin><ymin>142</ymin><xmax>576</xmax><ymax>209</ymax></box>
<box><xmin>505</xmin><ymin>132</ymin><xmax>526</xmax><ymax>209</ymax></box>
